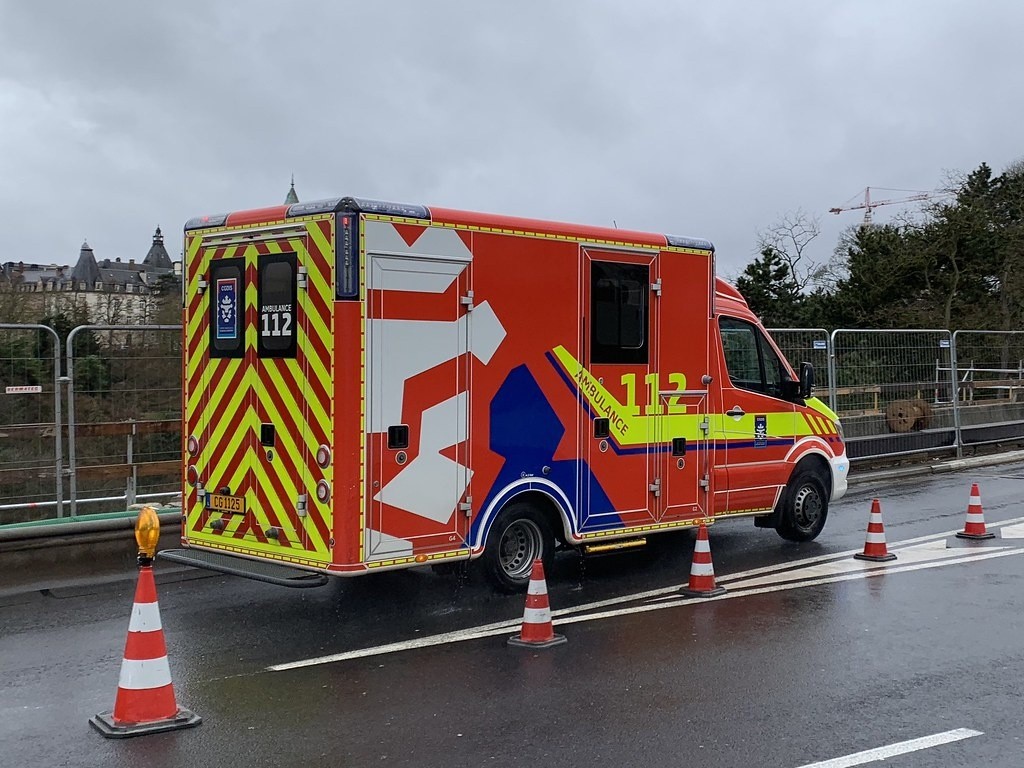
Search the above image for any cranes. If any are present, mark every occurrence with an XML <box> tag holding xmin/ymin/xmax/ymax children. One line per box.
<box><xmin>828</xmin><ymin>186</ymin><xmax>959</xmax><ymax>230</ymax></box>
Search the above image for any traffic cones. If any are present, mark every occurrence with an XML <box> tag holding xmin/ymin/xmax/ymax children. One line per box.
<box><xmin>86</xmin><ymin>507</ymin><xmax>204</xmax><ymax>740</ymax></box>
<box><xmin>679</xmin><ymin>522</ymin><xmax>727</xmax><ymax>598</ymax></box>
<box><xmin>853</xmin><ymin>498</ymin><xmax>899</xmax><ymax>562</ymax></box>
<box><xmin>508</xmin><ymin>558</ymin><xmax>568</xmax><ymax>649</ymax></box>
<box><xmin>955</xmin><ymin>483</ymin><xmax>995</xmax><ymax>541</ymax></box>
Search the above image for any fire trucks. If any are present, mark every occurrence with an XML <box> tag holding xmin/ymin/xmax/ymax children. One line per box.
<box><xmin>154</xmin><ymin>196</ymin><xmax>849</xmax><ymax>597</ymax></box>
